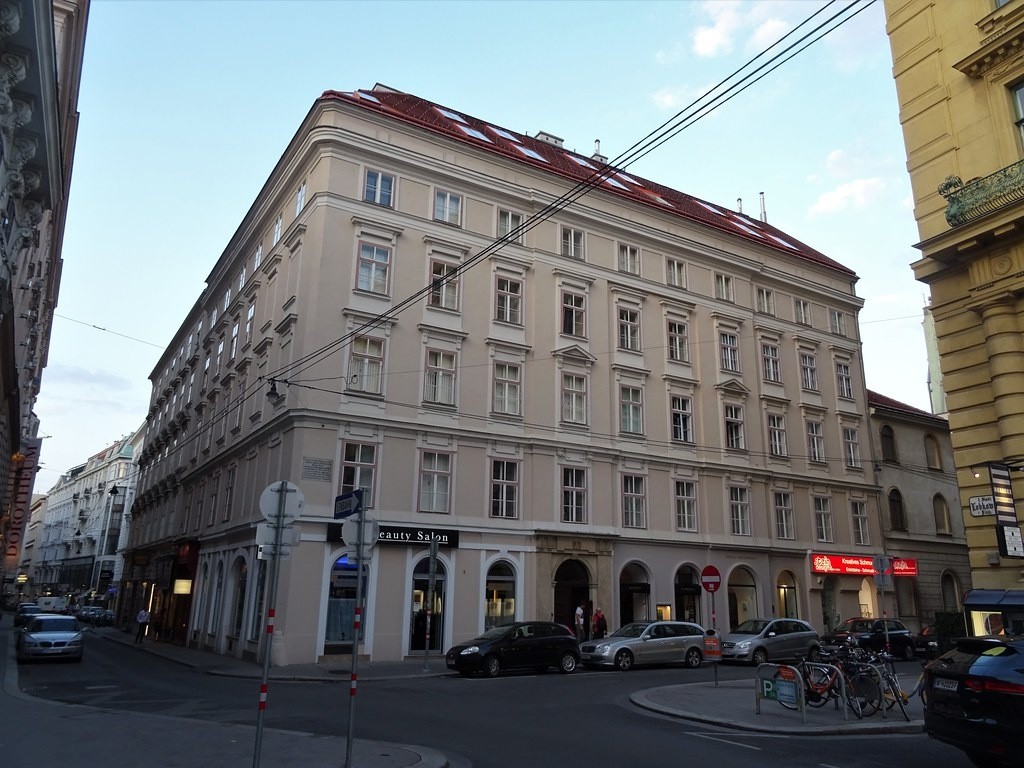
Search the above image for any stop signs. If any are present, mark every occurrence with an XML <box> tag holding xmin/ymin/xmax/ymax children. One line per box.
<box><xmin>701</xmin><ymin>565</ymin><xmax>721</xmax><ymax>593</ymax></box>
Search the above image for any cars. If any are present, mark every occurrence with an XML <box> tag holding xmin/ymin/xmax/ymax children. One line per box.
<box><xmin>67</xmin><ymin>604</ymin><xmax>118</xmax><ymax>626</ymax></box>
<box><xmin>13</xmin><ymin>602</ymin><xmax>64</xmax><ymax>652</ymax></box>
<box><xmin>720</xmin><ymin>617</ymin><xmax>821</xmax><ymax>668</ymax></box>
<box><xmin>15</xmin><ymin>614</ymin><xmax>90</xmax><ymax>666</ymax></box>
<box><xmin>579</xmin><ymin>621</ymin><xmax>707</xmax><ymax>672</ymax></box>
<box><xmin>821</xmin><ymin>616</ymin><xmax>912</xmax><ymax>661</ymax></box>
<box><xmin>444</xmin><ymin>619</ymin><xmax>582</xmax><ymax>679</ymax></box>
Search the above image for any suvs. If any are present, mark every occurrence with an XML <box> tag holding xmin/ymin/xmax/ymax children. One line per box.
<box><xmin>919</xmin><ymin>633</ymin><xmax>1024</xmax><ymax>768</ymax></box>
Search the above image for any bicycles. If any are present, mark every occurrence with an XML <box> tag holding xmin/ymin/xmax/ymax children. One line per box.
<box><xmin>774</xmin><ymin>636</ymin><xmax>927</xmax><ymax>723</ymax></box>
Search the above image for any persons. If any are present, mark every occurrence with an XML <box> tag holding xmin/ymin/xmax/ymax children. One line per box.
<box><xmin>134</xmin><ymin>604</ymin><xmax>150</xmax><ymax>645</ymax></box>
<box><xmin>823</xmin><ymin>612</ymin><xmax>830</xmax><ymax>635</ymax></box>
<box><xmin>3</xmin><ymin>589</ymin><xmax>80</xmax><ymax>617</ymax></box>
<box><xmin>575</xmin><ymin>600</ymin><xmax>587</xmax><ymax>645</ymax></box>
<box><xmin>592</xmin><ymin>607</ymin><xmax>607</xmax><ymax>640</ymax></box>
<box><xmin>918</xmin><ymin>623</ymin><xmax>929</xmax><ymax>648</ymax></box>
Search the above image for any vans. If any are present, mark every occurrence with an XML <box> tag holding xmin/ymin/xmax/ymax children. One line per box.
<box><xmin>35</xmin><ymin>596</ymin><xmax>68</xmax><ymax>614</ymax></box>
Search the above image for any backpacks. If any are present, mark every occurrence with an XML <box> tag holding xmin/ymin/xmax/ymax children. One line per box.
<box><xmin>595</xmin><ymin>613</ymin><xmax>604</xmax><ymax>632</ymax></box>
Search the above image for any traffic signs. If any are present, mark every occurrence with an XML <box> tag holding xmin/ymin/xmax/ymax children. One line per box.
<box><xmin>333</xmin><ymin>488</ymin><xmax>363</xmax><ymax>520</ymax></box>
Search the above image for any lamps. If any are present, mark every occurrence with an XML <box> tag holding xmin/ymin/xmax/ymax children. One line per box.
<box><xmin>265</xmin><ymin>380</ymin><xmax>280</xmax><ymax>406</ymax></box>
